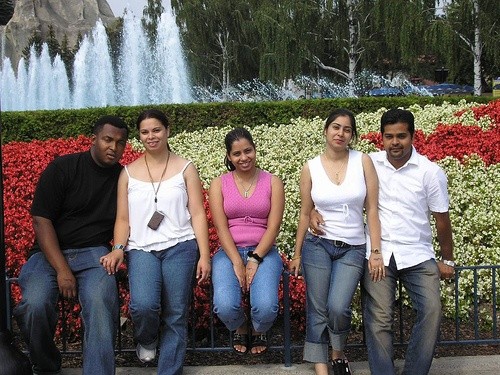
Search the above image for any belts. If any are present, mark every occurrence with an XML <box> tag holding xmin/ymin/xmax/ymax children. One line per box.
<box><xmin>325</xmin><ymin>239</ymin><xmax>360</xmax><ymax>248</ymax></box>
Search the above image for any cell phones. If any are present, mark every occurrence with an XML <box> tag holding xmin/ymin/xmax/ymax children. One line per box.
<box><xmin>148</xmin><ymin>210</ymin><xmax>165</xmax><ymax>230</ymax></box>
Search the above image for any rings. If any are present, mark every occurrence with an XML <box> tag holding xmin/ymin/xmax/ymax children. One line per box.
<box><xmin>249</xmin><ymin>277</ymin><xmax>250</xmax><ymax>278</ymax></box>
<box><xmin>379</xmin><ymin>268</ymin><xmax>381</xmax><ymax>270</ymax></box>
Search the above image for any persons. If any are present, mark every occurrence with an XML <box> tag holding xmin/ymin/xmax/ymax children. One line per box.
<box><xmin>309</xmin><ymin>109</ymin><xmax>456</xmax><ymax>375</ymax></box>
<box><xmin>18</xmin><ymin>114</ymin><xmax>128</xmax><ymax>375</ymax></box>
<box><xmin>99</xmin><ymin>110</ymin><xmax>211</xmax><ymax>374</ymax></box>
<box><xmin>289</xmin><ymin>109</ymin><xmax>387</xmax><ymax>374</ymax></box>
<box><xmin>210</xmin><ymin>128</ymin><xmax>285</xmax><ymax>357</ymax></box>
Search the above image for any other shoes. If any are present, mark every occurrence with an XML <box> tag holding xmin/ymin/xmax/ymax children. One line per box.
<box><xmin>136</xmin><ymin>342</ymin><xmax>157</xmax><ymax>366</ymax></box>
<box><xmin>331</xmin><ymin>354</ymin><xmax>352</xmax><ymax>375</ymax></box>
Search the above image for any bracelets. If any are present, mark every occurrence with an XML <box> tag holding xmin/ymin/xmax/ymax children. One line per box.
<box><xmin>247</xmin><ymin>251</ymin><xmax>263</xmax><ymax>264</ymax></box>
<box><xmin>112</xmin><ymin>245</ymin><xmax>125</xmax><ymax>250</ymax></box>
<box><xmin>292</xmin><ymin>256</ymin><xmax>301</xmax><ymax>259</ymax></box>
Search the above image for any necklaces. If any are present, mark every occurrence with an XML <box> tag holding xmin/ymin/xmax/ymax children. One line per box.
<box><xmin>235</xmin><ymin>173</ymin><xmax>252</xmax><ymax>197</ymax></box>
<box><xmin>324</xmin><ymin>154</ymin><xmax>342</xmax><ymax>185</ymax></box>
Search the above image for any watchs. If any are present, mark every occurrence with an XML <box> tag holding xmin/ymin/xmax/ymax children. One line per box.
<box><xmin>442</xmin><ymin>260</ymin><xmax>454</xmax><ymax>267</ymax></box>
<box><xmin>371</xmin><ymin>249</ymin><xmax>381</xmax><ymax>254</ymax></box>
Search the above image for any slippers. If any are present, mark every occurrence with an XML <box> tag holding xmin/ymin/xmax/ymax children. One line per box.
<box><xmin>250</xmin><ymin>324</ymin><xmax>269</xmax><ymax>357</ymax></box>
<box><xmin>232</xmin><ymin>321</ymin><xmax>250</xmax><ymax>356</ymax></box>
